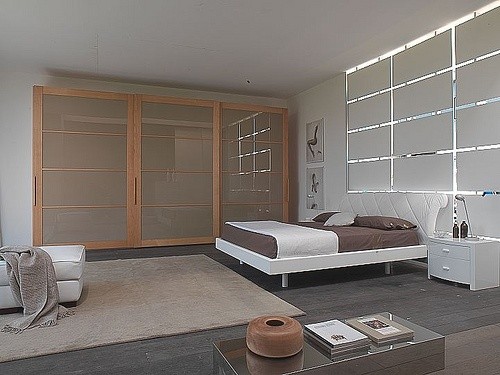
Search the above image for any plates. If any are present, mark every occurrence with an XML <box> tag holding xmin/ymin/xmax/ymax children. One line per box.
<box><xmin>434</xmin><ymin>228</ymin><xmax>450</xmax><ymax>237</ymax></box>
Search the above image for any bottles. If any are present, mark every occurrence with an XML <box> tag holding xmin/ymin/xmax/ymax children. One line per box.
<box><xmin>461</xmin><ymin>221</ymin><xmax>468</xmax><ymax>237</ymax></box>
<box><xmin>453</xmin><ymin>223</ymin><xmax>459</xmax><ymax>238</ymax></box>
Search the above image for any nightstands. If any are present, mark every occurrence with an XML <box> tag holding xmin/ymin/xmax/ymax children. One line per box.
<box><xmin>425</xmin><ymin>233</ymin><xmax>500</xmax><ymax>291</ymax></box>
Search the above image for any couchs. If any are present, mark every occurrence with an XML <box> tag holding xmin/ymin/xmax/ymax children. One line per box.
<box><xmin>0</xmin><ymin>243</ymin><xmax>86</xmax><ymax>311</ymax></box>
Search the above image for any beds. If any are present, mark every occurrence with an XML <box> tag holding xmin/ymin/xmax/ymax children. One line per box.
<box><xmin>215</xmin><ymin>191</ymin><xmax>448</xmax><ymax>289</ymax></box>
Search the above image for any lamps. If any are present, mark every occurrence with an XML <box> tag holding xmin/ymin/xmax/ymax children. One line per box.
<box><xmin>454</xmin><ymin>193</ymin><xmax>481</xmax><ymax>242</ymax></box>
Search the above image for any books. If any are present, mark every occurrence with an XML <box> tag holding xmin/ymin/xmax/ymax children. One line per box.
<box><xmin>345</xmin><ymin>314</ymin><xmax>415</xmax><ymax>344</ymax></box>
<box><xmin>303</xmin><ymin>320</ymin><xmax>372</xmax><ymax>354</ymax></box>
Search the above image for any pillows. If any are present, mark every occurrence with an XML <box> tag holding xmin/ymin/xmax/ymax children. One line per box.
<box><xmin>311</xmin><ymin>212</ymin><xmax>339</xmax><ymax>224</ymax></box>
<box><xmin>352</xmin><ymin>215</ymin><xmax>418</xmax><ymax>231</ymax></box>
<box><xmin>322</xmin><ymin>212</ymin><xmax>359</xmax><ymax>228</ymax></box>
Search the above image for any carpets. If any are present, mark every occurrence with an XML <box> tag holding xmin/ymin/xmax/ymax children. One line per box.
<box><xmin>0</xmin><ymin>252</ymin><xmax>307</xmax><ymax>364</ymax></box>
